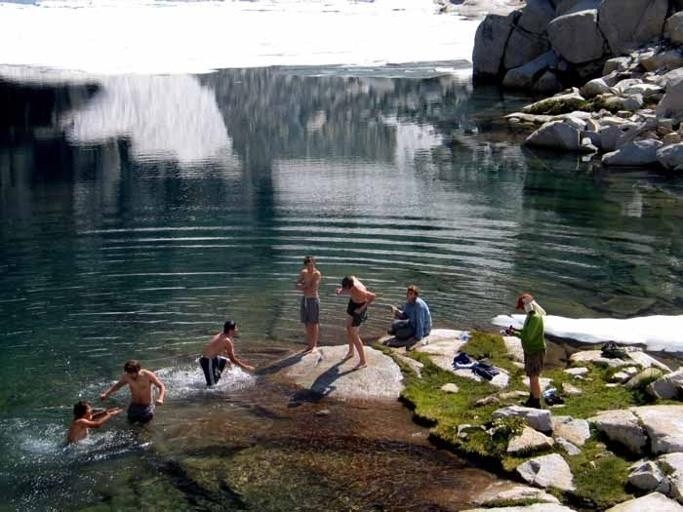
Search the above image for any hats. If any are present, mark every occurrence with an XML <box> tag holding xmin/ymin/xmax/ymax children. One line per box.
<box><xmin>516</xmin><ymin>294</ymin><xmax>534</xmax><ymax>309</ymax></box>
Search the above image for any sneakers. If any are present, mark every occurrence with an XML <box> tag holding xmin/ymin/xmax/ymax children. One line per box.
<box><xmin>545</xmin><ymin>393</ymin><xmax>564</xmax><ymax>405</ymax></box>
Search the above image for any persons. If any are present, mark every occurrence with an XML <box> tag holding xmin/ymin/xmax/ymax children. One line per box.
<box><xmin>99</xmin><ymin>359</ymin><xmax>166</xmax><ymax>428</ymax></box>
<box><xmin>66</xmin><ymin>401</ymin><xmax>124</xmax><ymax>446</ymax></box>
<box><xmin>504</xmin><ymin>292</ymin><xmax>547</xmax><ymax>410</ymax></box>
<box><xmin>292</xmin><ymin>254</ymin><xmax>322</xmax><ymax>355</ymax></box>
<box><xmin>197</xmin><ymin>319</ymin><xmax>256</xmax><ymax>387</ymax></box>
<box><xmin>334</xmin><ymin>273</ymin><xmax>377</xmax><ymax>370</ymax></box>
<box><xmin>384</xmin><ymin>284</ymin><xmax>433</xmax><ymax>342</ymax></box>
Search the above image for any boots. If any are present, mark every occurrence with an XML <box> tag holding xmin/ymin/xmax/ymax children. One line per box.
<box><xmin>520</xmin><ymin>392</ymin><xmax>541</xmax><ymax>408</ymax></box>
